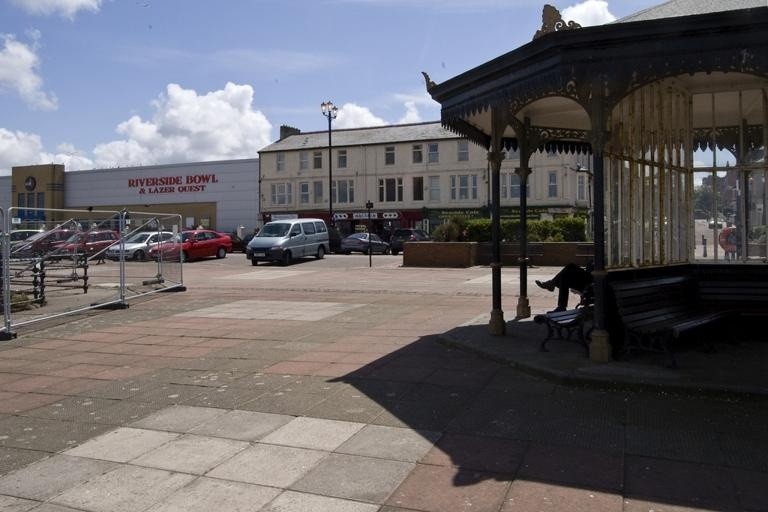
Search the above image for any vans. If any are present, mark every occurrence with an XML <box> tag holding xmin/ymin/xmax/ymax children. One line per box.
<box><xmin>390</xmin><ymin>227</ymin><xmax>431</xmax><ymax>253</ymax></box>
<box><xmin>243</xmin><ymin>217</ymin><xmax>331</xmax><ymax>267</ymax></box>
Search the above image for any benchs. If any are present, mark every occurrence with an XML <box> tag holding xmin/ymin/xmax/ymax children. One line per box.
<box><xmin>534</xmin><ymin>245</ymin><xmax>768</xmax><ymax>371</ymax></box>
<box><xmin>477</xmin><ymin>243</ymin><xmax>547</xmax><ymax>269</ymax></box>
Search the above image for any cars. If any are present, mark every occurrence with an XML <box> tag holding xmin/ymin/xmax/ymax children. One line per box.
<box><xmin>0</xmin><ymin>227</ymin><xmax>254</xmax><ymax>264</ymax></box>
<box><xmin>709</xmin><ymin>218</ymin><xmax>723</xmax><ymax>228</ymax></box>
<box><xmin>651</xmin><ymin>214</ymin><xmax>669</xmax><ymax>230</ymax></box>
<box><xmin>341</xmin><ymin>233</ymin><xmax>391</xmax><ymax>255</ymax></box>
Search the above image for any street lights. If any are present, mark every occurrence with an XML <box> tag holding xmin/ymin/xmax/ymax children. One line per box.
<box><xmin>319</xmin><ymin>101</ymin><xmax>338</xmax><ymax>255</ymax></box>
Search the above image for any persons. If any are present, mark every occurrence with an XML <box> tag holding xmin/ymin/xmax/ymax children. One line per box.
<box><xmin>535</xmin><ymin>240</ymin><xmax>619</xmax><ymax>314</ymax></box>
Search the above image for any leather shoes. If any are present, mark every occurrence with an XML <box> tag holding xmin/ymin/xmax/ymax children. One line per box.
<box><xmin>535</xmin><ymin>280</ymin><xmax>555</xmax><ymax>292</ymax></box>
<box><xmin>546</xmin><ymin>306</ymin><xmax>567</xmax><ymax>314</ymax></box>
